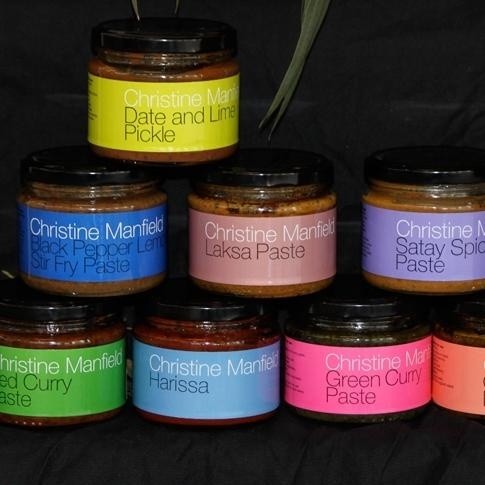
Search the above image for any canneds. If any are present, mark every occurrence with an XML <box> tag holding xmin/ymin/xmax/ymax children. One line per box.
<box><xmin>130</xmin><ymin>277</ymin><xmax>281</xmax><ymax>429</ymax></box>
<box><xmin>86</xmin><ymin>17</ymin><xmax>241</xmax><ymax>170</ymax></box>
<box><xmin>186</xmin><ymin>146</ymin><xmax>338</xmax><ymax>298</ymax></box>
<box><xmin>15</xmin><ymin>144</ymin><xmax>168</xmax><ymax>299</ymax></box>
<box><xmin>360</xmin><ymin>145</ymin><xmax>484</xmax><ymax>297</ymax></box>
<box><xmin>0</xmin><ymin>278</ymin><xmax>127</xmax><ymax>432</ymax></box>
<box><xmin>432</xmin><ymin>300</ymin><xmax>484</xmax><ymax>421</ymax></box>
<box><xmin>283</xmin><ymin>271</ymin><xmax>432</xmax><ymax>430</ymax></box>
